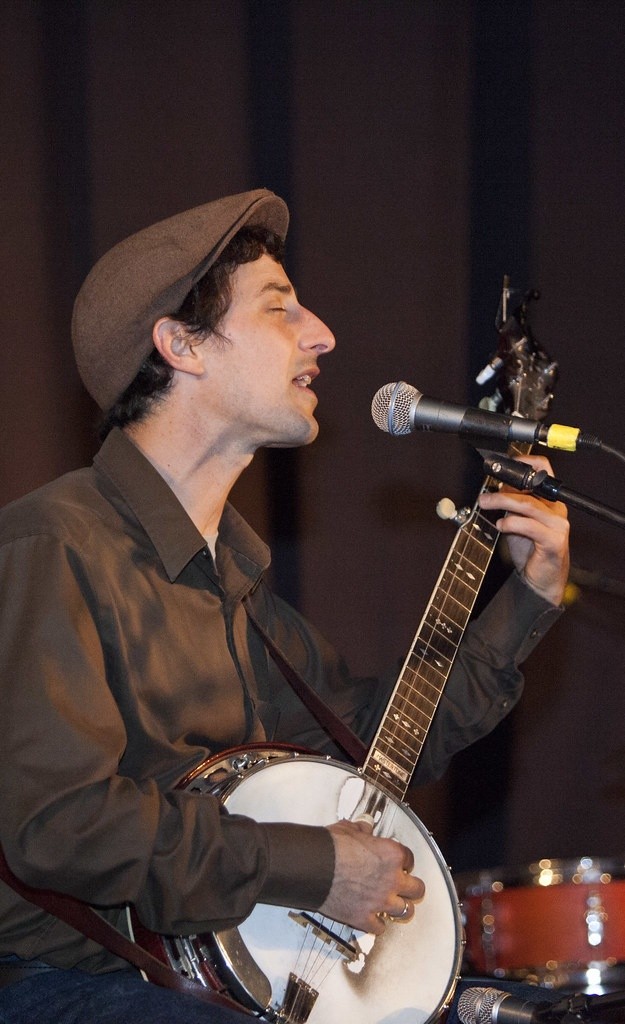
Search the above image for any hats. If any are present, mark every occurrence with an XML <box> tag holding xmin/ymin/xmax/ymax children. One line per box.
<box><xmin>71</xmin><ymin>188</ymin><xmax>289</xmax><ymax>413</ymax></box>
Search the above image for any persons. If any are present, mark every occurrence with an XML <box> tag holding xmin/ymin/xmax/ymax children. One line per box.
<box><xmin>0</xmin><ymin>188</ymin><xmax>572</xmax><ymax>1024</ymax></box>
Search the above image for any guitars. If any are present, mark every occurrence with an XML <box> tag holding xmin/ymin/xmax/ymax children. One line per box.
<box><xmin>125</xmin><ymin>260</ymin><xmax>560</xmax><ymax>1023</ymax></box>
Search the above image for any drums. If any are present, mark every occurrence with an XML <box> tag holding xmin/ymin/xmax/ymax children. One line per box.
<box><xmin>455</xmin><ymin>854</ymin><xmax>623</xmax><ymax>989</ymax></box>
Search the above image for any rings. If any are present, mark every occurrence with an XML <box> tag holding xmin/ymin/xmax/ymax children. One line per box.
<box><xmin>391</xmin><ymin>899</ymin><xmax>409</xmax><ymax>918</ymax></box>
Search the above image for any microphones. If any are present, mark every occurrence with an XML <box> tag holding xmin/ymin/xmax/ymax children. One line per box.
<box><xmin>371</xmin><ymin>381</ymin><xmax>581</xmax><ymax>453</ymax></box>
<box><xmin>457</xmin><ymin>986</ymin><xmax>615</xmax><ymax>1024</ymax></box>
